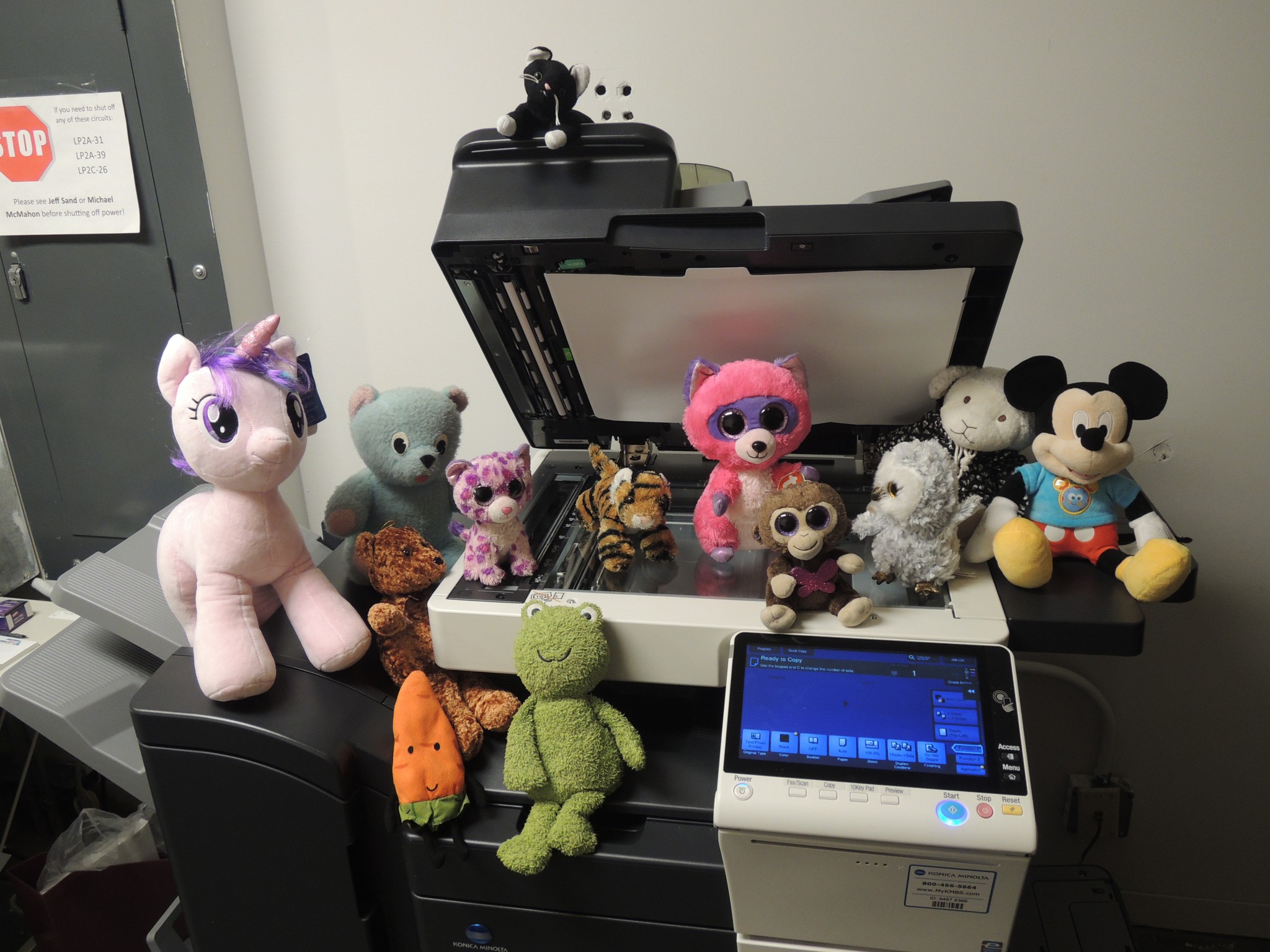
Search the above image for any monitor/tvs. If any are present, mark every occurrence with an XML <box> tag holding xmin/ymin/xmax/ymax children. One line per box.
<box><xmin>709</xmin><ymin>630</ymin><xmax>1036</xmax><ymax>854</ymax></box>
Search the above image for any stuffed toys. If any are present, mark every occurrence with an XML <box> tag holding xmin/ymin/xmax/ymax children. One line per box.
<box><xmin>758</xmin><ymin>481</ymin><xmax>872</xmax><ymax>631</ymax></box>
<box><xmin>155</xmin><ymin>312</ymin><xmax>372</xmax><ymax>701</ymax></box>
<box><xmin>683</xmin><ymin>351</ymin><xmax>818</xmax><ymax>562</ymax></box>
<box><xmin>575</xmin><ymin>443</ymin><xmax>678</xmax><ymax>572</ymax></box>
<box><xmin>326</xmin><ymin>383</ymin><xmax>467</xmax><ymax>579</ymax></box>
<box><xmin>879</xmin><ymin>364</ymin><xmax>1029</xmax><ymax>547</ymax></box>
<box><xmin>965</xmin><ymin>356</ymin><xmax>1191</xmax><ymax>602</ymax></box>
<box><xmin>444</xmin><ymin>443</ymin><xmax>538</xmax><ymax>587</ymax></box>
<box><xmin>353</xmin><ymin>521</ymin><xmax>523</xmax><ymax>760</ymax></box>
<box><xmin>389</xmin><ymin>672</ymin><xmax>469</xmax><ymax>836</ymax></box>
<box><xmin>494</xmin><ymin>599</ymin><xmax>644</xmax><ymax>876</ymax></box>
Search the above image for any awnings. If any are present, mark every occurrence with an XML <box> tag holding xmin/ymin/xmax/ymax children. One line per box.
<box><xmin>849</xmin><ymin>437</ymin><xmax>983</xmax><ymax>594</ymax></box>
